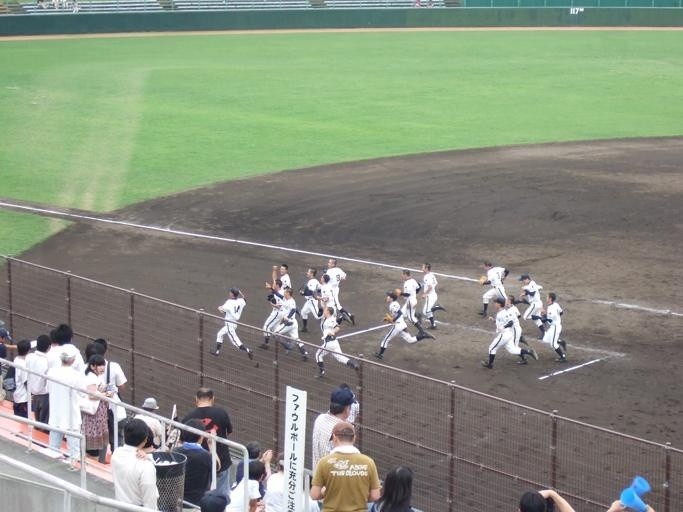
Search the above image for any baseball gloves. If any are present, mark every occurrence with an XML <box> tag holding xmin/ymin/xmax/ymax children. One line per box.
<box><xmin>382</xmin><ymin>313</ymin><xmax>394</xmax><ymax>323</ymax></box>
<box><xmin>479</xmin><ymin>276</ymin><xmax>487</xmax><ymax>285</ymax></box>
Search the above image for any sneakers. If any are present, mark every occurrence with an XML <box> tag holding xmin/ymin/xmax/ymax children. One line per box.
<box><xmin>478</xmin><ymin>312</ymin><xmax>487</xmax><ymax>316</ymax></box>
<box><xmin>313</xmin><ymin>370</ymin><xmax>324</xmax><ymax>379</ymax></box>
<box><xmin>375</xmin><ymin>353</ymin><xmax>383</xmax><ymax>359</ymax></box>
<box><xmin>296</xmin><ymin>305</ymin><xmax>300</xmax><ymax>317</ymax></box>
<box><xmin>248</xmin><ymin>350</ymin><xmax>253</xmax><ymax>359</ymax></box>
<box><xmin>415</xmin><ymin>303</ymin><xmax>446</xmax><ymax>340</ymax></box>
<box><xmin>257</xmin><ymin>344</ymin><xmax>268</xmax><ymax>349</ymax></box>
<box><xmin>481</xmin><ymin>360</ymin><xmax>492</xmax><ymax>369</ymax></box>
<box><xmin>556</xmin><ymin>340</ymin><xmax>568</xmax><ymax>361</ymax></box>
<box><xmin>341</xmin><ymin>312</ymin><xmax>354</xmax><ymax>325</ymax></box>
<box><xmin>209</xmin><ymin>348</ymin><xmax>218</xmax><ymax>356</ymax></box>
<box><xmin>516</xmin><ymin>336</ymin><xmax>539</xmax><ymax>365</ymax></box>
<box><xmin>299</xmin><ymin>328</ymin><xmax>307</xmax><ymax>333</ymax></box>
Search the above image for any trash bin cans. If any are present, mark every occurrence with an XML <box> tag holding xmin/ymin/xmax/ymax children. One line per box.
<box><xmin>147</xmin><ymin>452</ymin><xmax>187</xmax><ymax>512</ymax></box>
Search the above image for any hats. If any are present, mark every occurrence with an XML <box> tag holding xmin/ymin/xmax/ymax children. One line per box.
<box><xmin>329</xmin><ymin>421</ymin><xmax>354</xmax><ymax>441</ymax></box>
<box><xmin>142</xmin><ymin>397</ymin><xmax>159</xmax><ymax>410</ymax></box>
<box><xmin>0</xmin><ymin>328</ymin><xmax>12</xmax><ymax>342</ymax></box>
<box><xmin>331</xmin><ymin>388</ymin><xmax>356</xmax><ymax>406</ymax></box>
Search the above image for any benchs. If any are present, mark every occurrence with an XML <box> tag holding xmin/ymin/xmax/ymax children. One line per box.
<box><xmin>18</xmin><ymin>0</ymin><xmax>451</xmax><ymax>16</ymax></box>
